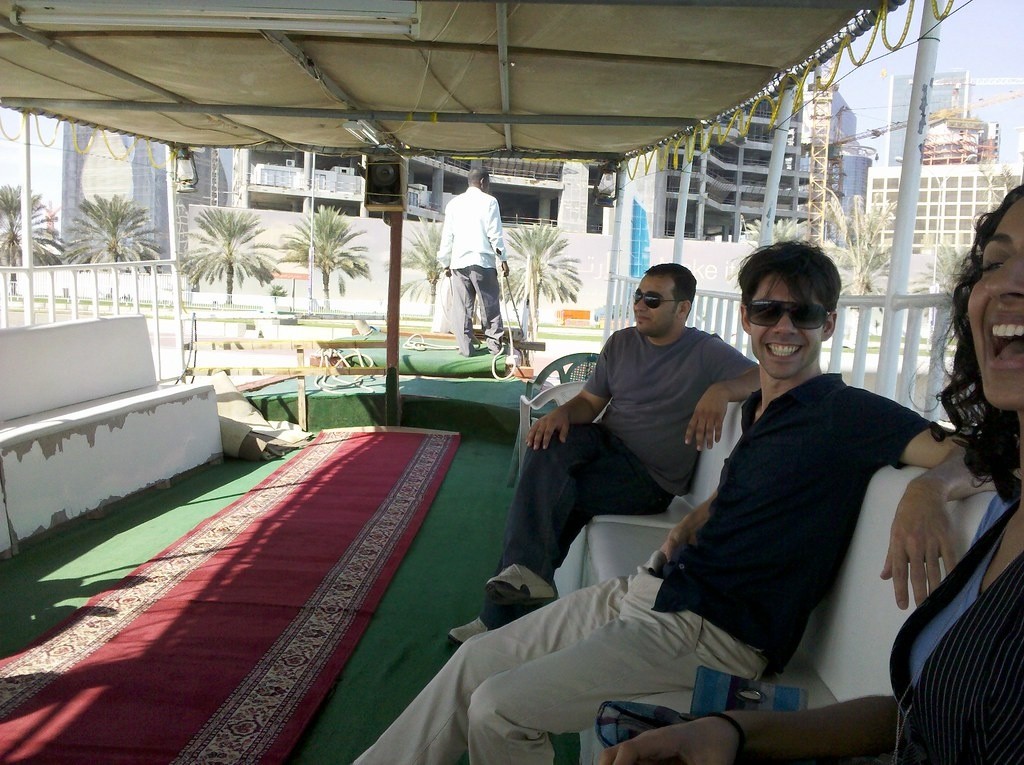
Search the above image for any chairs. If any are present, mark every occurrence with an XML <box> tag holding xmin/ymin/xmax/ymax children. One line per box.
<box><xmin>507</xmin><ymin>353</ymin><xmax>599</xmax><ymax>488</ymax></box>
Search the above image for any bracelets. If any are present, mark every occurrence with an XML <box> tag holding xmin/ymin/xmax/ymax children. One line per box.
<box><xmin>704</xmin><ymin>712</ymin><xmax>745</xmax><ymax>747</ymax></box>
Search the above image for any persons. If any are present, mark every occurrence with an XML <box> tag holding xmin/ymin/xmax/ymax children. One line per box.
<box><xmin>601</xmin><ymin>187</ymin><xmax>1024</xmax><ymax>765</ymax></box>
<box><xmin>352</xmin><ymin>240</ymin><xmax>996</xmax><ymax>765</ymax></box>
<box><xmin>437</xmin><ymin>168</ymin><xmax>509</xmax><ymax>358</ymax></box>
<box><xmin>448</xmin><ymin>264</ymin><xmax>762</xmax><ymax>645</ymax></box>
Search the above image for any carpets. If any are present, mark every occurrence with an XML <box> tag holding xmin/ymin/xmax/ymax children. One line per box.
<box><xmin>0</xmin><ymin>425</ymin><xmax>462</xmax><ymax>765</ymax></box>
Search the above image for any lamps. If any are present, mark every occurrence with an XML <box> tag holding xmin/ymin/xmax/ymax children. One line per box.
<box><xmin>592</xmin><ymin>161</ymin><xmax>619</xmax><ymax>208</ymax></box>
<box><xmin>174</xmin><ymin>143</ymin><xmax>198</xmax><ymax>194</ymax></box>
<box><xmin>286</xmin><ymin>159</ymin><xmax>295</xmax><ymax>167</ymax></box>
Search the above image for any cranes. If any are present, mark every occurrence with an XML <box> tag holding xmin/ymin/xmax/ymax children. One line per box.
<box><xmin>806</xmin><ymin>87</ymin><xmax>1024</xmax><ymax>242</ymax></box>
<box><xmin>908</xmin><ymin>74</ymin><xmax>1024</xmax><ymax>117</ymax></box>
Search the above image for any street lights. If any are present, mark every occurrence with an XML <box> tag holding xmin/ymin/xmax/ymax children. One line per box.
<box><xmin>894</xmin><ymin>154</ymin><xmax>980</xmax><ymax>351</ymax></box>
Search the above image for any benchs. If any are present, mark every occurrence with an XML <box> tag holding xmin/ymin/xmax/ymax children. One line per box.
<box><xmin>554</xmin><ymin>401</ymin><xmax>1013</xmax><ymax>765</ymax></box>
<box><xmin>1</xmin><ymin>314</ymin><xmax>223</xmax><ymax>564</ymax></box>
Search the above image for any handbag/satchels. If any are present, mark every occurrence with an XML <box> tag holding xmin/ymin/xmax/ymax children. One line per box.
<box><xmin>594</xmin><ymin>665</ymin><xmax>810</xmax><ymax>748</ymax></box>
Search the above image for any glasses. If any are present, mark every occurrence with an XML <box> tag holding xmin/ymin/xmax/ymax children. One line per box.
<box><xmin>632</xmin><ymin>288</ymin><xmax>675</xmax><ymax>309</ymax></box>
<box><xmin>746</xmin><ymin>299</ymin><xmax>831</xmax><ymax>330</ymax></box>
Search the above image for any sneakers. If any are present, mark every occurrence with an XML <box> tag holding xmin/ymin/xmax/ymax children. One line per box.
<box><xmin>448</xmin><ymin>615</ymin><xmax>499</xmax><ymax>645</ymax></box>
<box><xmin>484</xmin><ymin>564</ymin><xmax>560</xmax><ymax>608</ymax></box>
